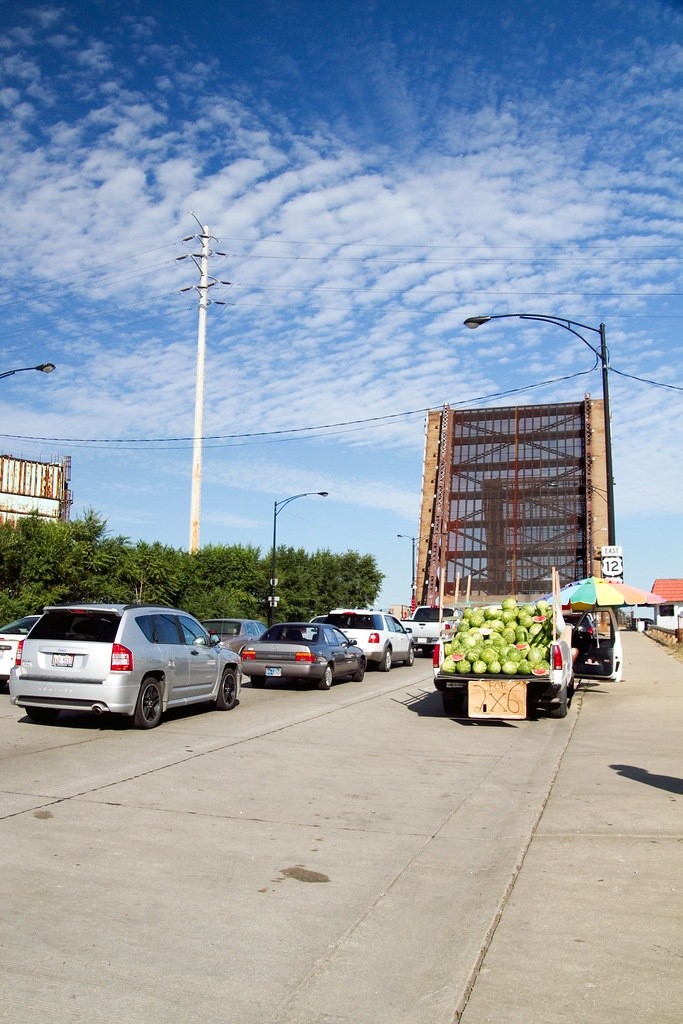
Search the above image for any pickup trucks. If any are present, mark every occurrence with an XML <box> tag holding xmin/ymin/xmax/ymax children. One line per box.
<box><xmin>431</xmin><ymin>601</ymin><xmax>623</xmax><ymax>719</ymax></box>
<box><xmin>400</xmin><ymin>606</ymin><xmax>454</xmax><ymax>657</ymax></box>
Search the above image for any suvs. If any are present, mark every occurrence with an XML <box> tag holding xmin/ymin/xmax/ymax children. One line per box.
<box><xmin>323</xmin><ymin>609</ymin><xmax>415</xmax><ymax>672</ymax></box>
<box><xmin>6</xmin><ymin>602</ymin><xmax>244</xmax><ymax>730</ymax></box>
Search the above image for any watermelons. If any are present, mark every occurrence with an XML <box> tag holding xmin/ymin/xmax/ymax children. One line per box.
<box><xmin>441</xmin><ymin>599</ymin><xmax>553</xmax><ymax>675</ymax></box>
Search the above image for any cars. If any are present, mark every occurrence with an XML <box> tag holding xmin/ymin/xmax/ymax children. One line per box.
<box><xmin>0</xmin><ymin>615</ymin><xmax>43</xmax><ymax>694</ymax></box>
<box><xmin>237</xmin><ymin>622</ymin><xmax>367</xmax><ymax>690</ymax></box>
<box><xmin>308</xmin><ymin>614</ymin><xmax>328</xmax><ymax>623</ymax></box>
<box><xmin>201</xmin><ymin>618</ymin><xmax>268</xmax><ymax>653</ymax></box>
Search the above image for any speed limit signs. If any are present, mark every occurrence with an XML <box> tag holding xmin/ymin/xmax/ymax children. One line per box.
<box><xmin>606</xmin><ymin>560</ymin><xmax>619</xmax><ymax>572</ymax></box>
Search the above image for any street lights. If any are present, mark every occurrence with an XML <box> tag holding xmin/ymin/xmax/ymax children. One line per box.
<box><xmin>465</xmin><ymin>310</ymin><xmax>624</xmax><ymax>581</ymax></box>
<box><xmin>268</xmin><ymin>490</ymin><xmax>330</xmax><ymax>628</ymax></box>
<box><xmin>398</xmin><ymin>534</ymin><xmax>418</xmax><ymax>597</ymax></box>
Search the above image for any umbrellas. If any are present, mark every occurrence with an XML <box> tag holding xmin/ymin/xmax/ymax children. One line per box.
<box><xmin>534</xmin><ymin>574</ymin><xmax>668</xmax><ymax>650</ymax></box>
